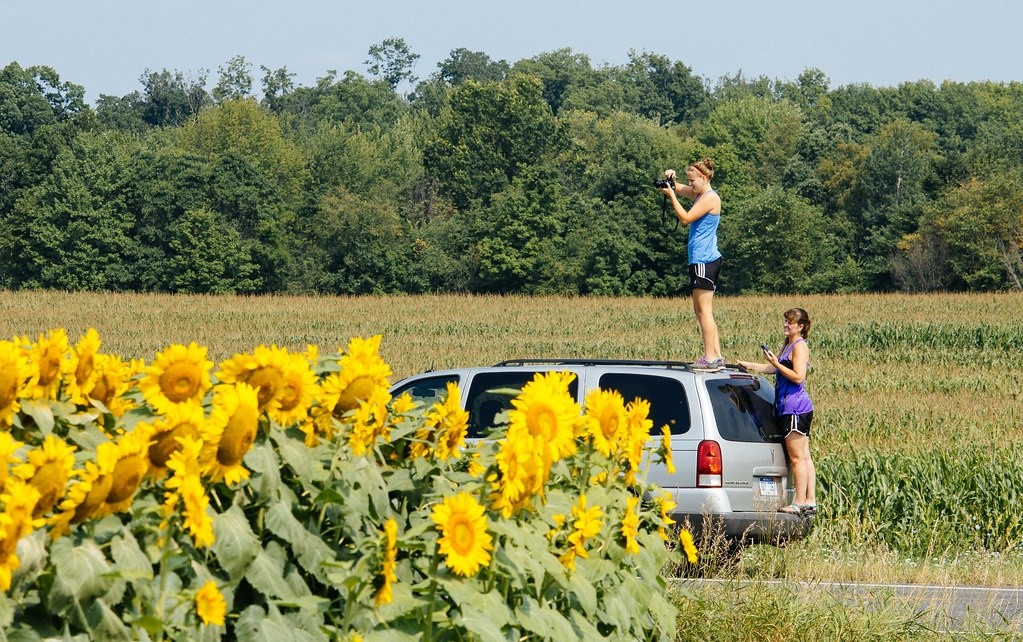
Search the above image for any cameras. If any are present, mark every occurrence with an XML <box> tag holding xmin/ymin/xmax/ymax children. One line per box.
<box><xmin>653</xmin><ymin>176</ymin><xmax>676</xmax><ymax>191</ymax></box>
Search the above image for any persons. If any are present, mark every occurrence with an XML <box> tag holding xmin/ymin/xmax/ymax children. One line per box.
<box><xmin>661</xmin><ymin>157</ymin><xmax>727</xmax><ymax>372</ymax></box>
<box><xmin>735</xmin><ymin>308</ymin><xmax>817</xmax><ymax>514</ymax></box>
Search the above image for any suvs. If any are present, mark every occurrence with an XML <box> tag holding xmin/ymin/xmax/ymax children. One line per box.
<box><xmin>383</xmin><ymin>355</ymin><xmax>818</xmax><ymax>583</ymax></box>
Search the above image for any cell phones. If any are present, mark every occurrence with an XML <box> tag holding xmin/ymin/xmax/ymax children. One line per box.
<box><xmin>761</xmin><ymin>345</ymin><xmax>768</xmax><ymax>353</ymax></box>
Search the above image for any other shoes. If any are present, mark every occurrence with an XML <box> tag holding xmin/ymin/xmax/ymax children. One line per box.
<box><xmin>777</xmin><ymin>500</ymin><xmax>818</xmax><ymax>513</ymax></box>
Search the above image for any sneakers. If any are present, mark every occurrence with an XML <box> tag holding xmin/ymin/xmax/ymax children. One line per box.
<box><xmin>688</xmin><ymin>354</ymin><xmax>726</xmax><ymax>372</ymax></box>
